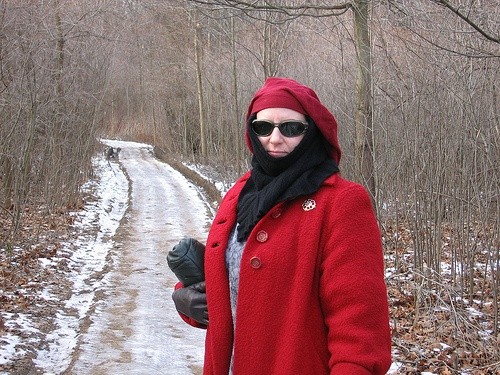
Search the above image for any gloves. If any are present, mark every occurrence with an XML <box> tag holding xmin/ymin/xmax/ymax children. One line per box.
<box><xmin>172</xmin><ymin>281</ymin><xmax>209</xmax><ymax>326</ymax></box>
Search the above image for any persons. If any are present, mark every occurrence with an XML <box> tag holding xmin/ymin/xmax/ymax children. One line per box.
<box><xmin>173</xmin><ymin>76</ymin><xmax>393</xmax><ymax>375</ymax></box>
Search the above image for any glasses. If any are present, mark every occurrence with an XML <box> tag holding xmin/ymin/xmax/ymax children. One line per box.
<box><xmin>251</xmin><ymin>119</ymin><xmax>309</xmax><ymax>138</ymax></box>
